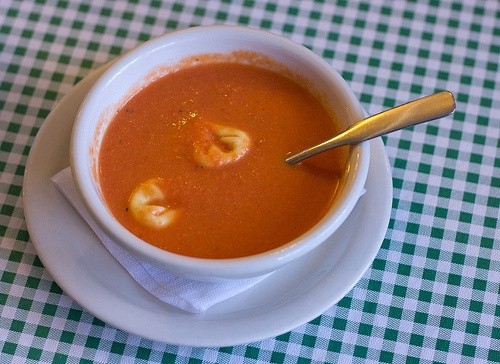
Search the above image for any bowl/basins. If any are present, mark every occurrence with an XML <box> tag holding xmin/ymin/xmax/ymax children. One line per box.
<box><xmin>69</xmin><ymin>24</ymin><xmax>370</xmax><ymax>277</ymax></box>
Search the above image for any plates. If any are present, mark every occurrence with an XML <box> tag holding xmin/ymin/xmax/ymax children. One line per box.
<box><xmin>21</xmin><ymin>54</ymin><xmax>393</xmax><ymax>348</ymax></box>
